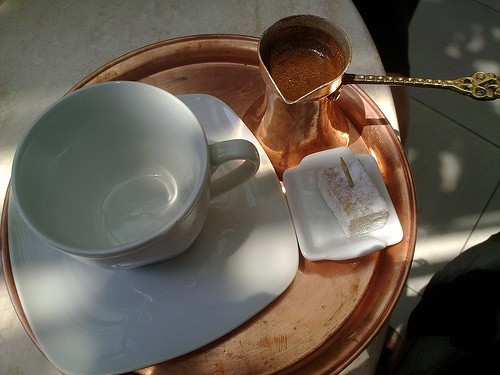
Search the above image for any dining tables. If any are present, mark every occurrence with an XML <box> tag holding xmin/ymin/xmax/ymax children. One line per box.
<box><xmin>0</xmin><ymin>0</ymin><xmax>401</xmax><ymax>375</ymax></box>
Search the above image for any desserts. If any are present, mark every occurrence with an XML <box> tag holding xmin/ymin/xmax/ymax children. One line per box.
<box><xmin>319</xmin><ymin>157</ymin><xmax>390</xmax><ymax>238</ymax></box>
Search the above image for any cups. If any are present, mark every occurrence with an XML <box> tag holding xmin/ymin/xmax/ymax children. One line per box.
<box><xmin>11</xmin><ymin>81</ymin><xmax>261</xmax><ymax>270</ymax></box>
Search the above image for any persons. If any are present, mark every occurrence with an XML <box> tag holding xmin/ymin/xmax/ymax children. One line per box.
<box><xmin>375</xmin><ymin>231</ymin><xmax>500</xmax><ymax>375</ymax></box>
<box><xmin>351</xmin><ymin>0</ymin><xmax>419</xmax><ymax>144</ymax></box>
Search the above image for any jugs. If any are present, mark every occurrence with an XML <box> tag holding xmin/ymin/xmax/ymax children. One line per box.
<box><xmin>256</xmin><ymin>14</ymin><xmax>500</xmax><ymax>181</ymax></box>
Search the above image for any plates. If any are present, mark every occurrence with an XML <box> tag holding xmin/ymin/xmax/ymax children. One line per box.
<box><xmin>8</xmin><ymin>94</ymin><xmax>300</xmax><ymax>375</ymax></box>
<box><xmin>282</xmin><ymin>146</ymin><xmax>403</xmax><ymax>261</ymax></box>
